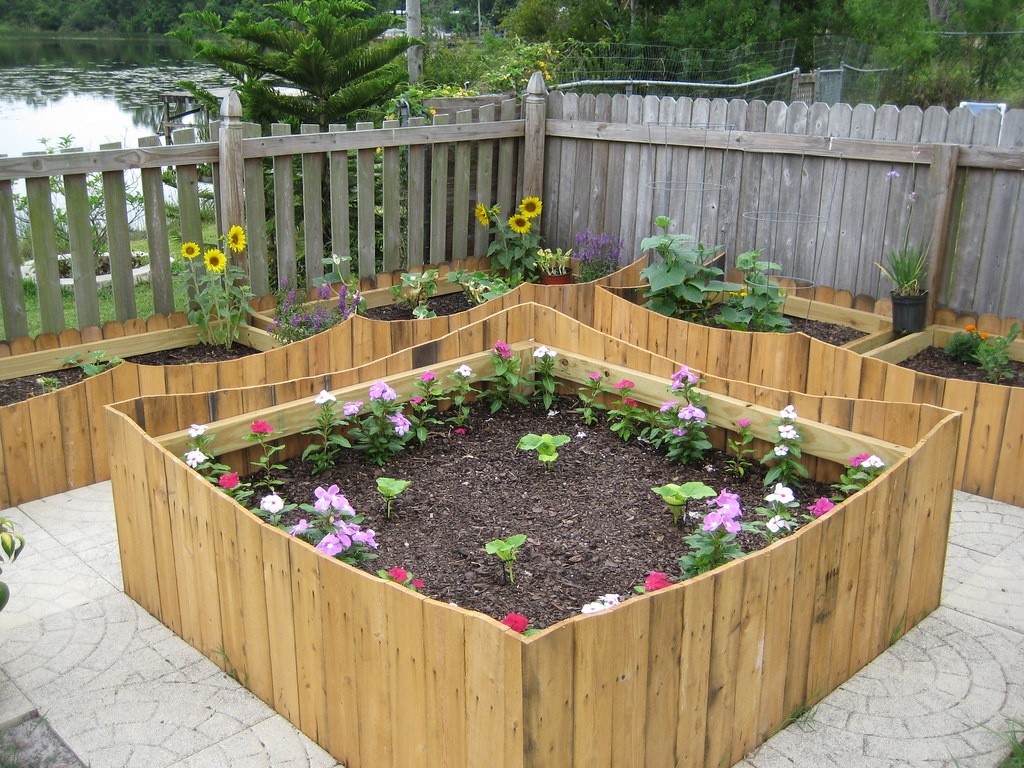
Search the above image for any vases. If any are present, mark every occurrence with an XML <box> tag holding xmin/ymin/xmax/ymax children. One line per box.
<box><xmin>246</xmin><ymin>252</ymin><xmax>648</xmax><ymax>367</ymax></box>
<box><xmin>806</xmin><ymin>310</ymin><xmax>1024</xmax><ymax>508</ymax></box>
<box><xmin>593</xmin><ymin>268</ymin><xmax>894</xmax><ymax>394</ymax></box>
<box><xmin>97</xmin><ymin>299</ymin><xmax>964</xmax><ymax>768</ymax></box>
<box><xmin>0</xmin><ymin>302</ymin><xmax>354</xmax><ymax>515</ymax></box>
<box><xmin>888</xmin><ymin>288</ymin><xmax>928</xmax><ymax>335</ymax></box>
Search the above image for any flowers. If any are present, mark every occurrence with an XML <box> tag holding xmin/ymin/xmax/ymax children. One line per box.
<box><xmin>946</xmin><ymin>323</ymin><xmax>988</xmax><ymax>364</ymax></box>
<box><xmin>179</xmin><ymin>340</ymin><xmax>888</xmax><ymax>641</ymax></box>
<box><xmin>636</xmin><ymin>215</ymin><xmax>743</xmax><ymax>324</ymax></box>
<box><xmin>871</xmin><ymin>145</ymin><xmax>937</xmax><ymax>296</ymax></box>
<box><xmin>173</xmin><ymin>226</ymin><xmax>256</xmax><ymax>353</ymax></box>
<box><xmin>475</xmin><ymin>195</ymin><xmax>546</xmax><ymax>282</ymax></box>
<box><xmin>713</xmin><ymin>248</ymin><xmax>794</xmax><ymax>333</ymax></box>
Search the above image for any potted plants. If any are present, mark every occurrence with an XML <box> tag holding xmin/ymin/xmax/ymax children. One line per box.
<box><xmin>532</xmin><ymin>247</ymin><xmax>574</xmax><ymax>284</ymax></box>
